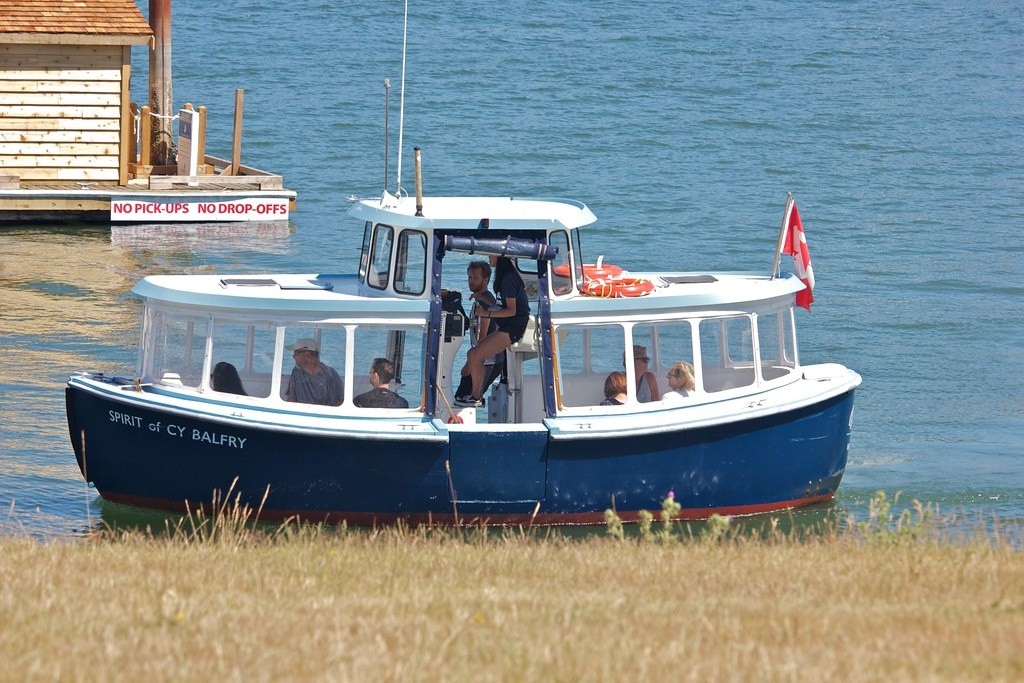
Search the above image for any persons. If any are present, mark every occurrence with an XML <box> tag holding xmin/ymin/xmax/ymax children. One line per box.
<box><xmin>623</xmin><ymin>345</ymin><xmax>659</xmax><ymax>403</ymax></box>
<box><xmin>284</xmin><ymin>338</ymin><xmax>344</xmax><ymax>406</ymax></box>
<box><xmin>454</xmin><ymin>252</ymin><xmax>531</xmax><ymax>408</ymax></box>
<box><xmin>353</xmin><ymin>358</ymin><xmax>409</xmax><ymax>408</ymax></box>
<box><xmin>600</xmin><ymin>372</ymin><xmax>626</xmax><ymax>405</ymax></box>
<box><xmin>662</xmin><ymin>361</ymin><xmax>695</xmax><ymax>400</ymax></box>
<box><xmin>211</xmin><ymin>362</ymin><xmax>248</xmax><ymax>395</ymax></box>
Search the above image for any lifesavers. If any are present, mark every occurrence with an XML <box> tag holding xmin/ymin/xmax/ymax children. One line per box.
<box><xmin>580</xmin><ymin>278</ymin><xmax>654</xmax><ymax>297</ymax></box>
<box><xmin>554</xmin><ymin>263</ymin><xmax>622</xmax><ymax>279</ymax></box>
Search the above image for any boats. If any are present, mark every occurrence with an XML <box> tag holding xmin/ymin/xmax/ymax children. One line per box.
<box><xmin>65</xmin><ymin>1</ymin><xmax>864</xmax><ymax>534</ymax></box>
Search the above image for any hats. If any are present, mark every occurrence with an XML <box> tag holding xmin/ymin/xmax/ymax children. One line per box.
<box><xmin>285</xmin><ymin>338</ymin><xmax>320</xmax><ymax>353</ymax></box>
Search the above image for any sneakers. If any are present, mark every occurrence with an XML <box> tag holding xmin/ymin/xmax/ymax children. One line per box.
<box><xmin>455</xmin><ymin>394</ymin><xmax>485</xmax><ymax>409</ymax></box>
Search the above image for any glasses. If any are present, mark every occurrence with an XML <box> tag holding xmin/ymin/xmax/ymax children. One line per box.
<box><xmin>666</xmin><ymin>373</ymin><xmax>677</xmax><ymax>378</ymax></box>
<box><xmin>210</xmin><ymin>374</ymin><xmax>215</xmax><ymax>379</ymax></box>
<box><xmin>634</xmin><ymin>357</ymin><xmax>650</xmax><ymax>363</ymax></box>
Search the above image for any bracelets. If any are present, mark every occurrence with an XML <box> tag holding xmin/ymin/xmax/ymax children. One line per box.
<box><xmin>488</xmin><ymin>311</ymin><xmax>491</xmax><ymax>318</ymax></box>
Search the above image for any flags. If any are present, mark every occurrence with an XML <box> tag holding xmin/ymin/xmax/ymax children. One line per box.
<box><xmin>780</xmin><ymin>198</ymin><xmax>815</xmax><ymax>313</ymax></box>
